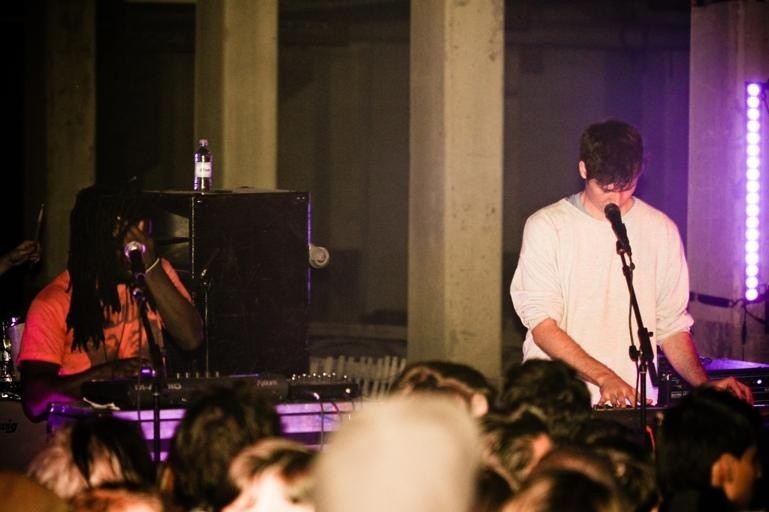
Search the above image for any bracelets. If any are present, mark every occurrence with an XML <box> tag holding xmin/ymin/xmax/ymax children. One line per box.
<box><xmin>145</xmin><ymin>257</ymin><xmax>160</xmax><ymax>274</ymax></box>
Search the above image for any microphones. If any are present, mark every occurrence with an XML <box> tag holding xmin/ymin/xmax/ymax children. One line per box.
<box><xmin>123</xmin><ymin>240</ymin><xmax>147</xmax><ymax>284</ymax></box>
<box><xmin>604</xmin><ymin>202</ymin><xmax>633</xmax><ymax>257</ymax></box>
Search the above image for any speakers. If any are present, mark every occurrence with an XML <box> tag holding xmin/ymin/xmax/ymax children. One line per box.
<box><xmin>139</xmin><ymin>186</ymin><xmax>311</xmax><ymax>379</ymax></box>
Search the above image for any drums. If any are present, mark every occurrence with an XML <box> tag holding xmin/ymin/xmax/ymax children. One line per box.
<box><xmin>0</xmin><ymin>317</ymin><xmax>28</xmax><ymax>401</ymax></box>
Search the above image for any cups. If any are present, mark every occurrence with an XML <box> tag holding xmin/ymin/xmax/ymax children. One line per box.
<box><xmin>193</xmin><ymin>138</ymin><xmax>213</xmax><ymax>192</ymax></box>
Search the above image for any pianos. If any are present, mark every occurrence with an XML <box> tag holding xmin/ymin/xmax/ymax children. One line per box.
<box><xmin>594</xmin><ymin>395</ymin><xmax>769</xmax><ymax>428</ymax></box>
<box><xmin>70</xmin><ymin>369</ymin><xmax>362</xmax><ymax>412</ymax></box>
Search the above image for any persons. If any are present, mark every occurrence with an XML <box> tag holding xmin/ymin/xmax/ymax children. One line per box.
<box><xmin>16</xmin><ymin>186</ymin><xmax>205</xmax><ymax>424</ymax></box>
<box><xmin>317</xmin><ymin>358</ymin><xmax>769</xmax><ymax>511</ymax></box>
<box><xmin>2</xmin><ymin>388</ymin><xmax>319</xmax><ymax>512</ymax></box>
<box><xmin>0</xmin><ymin>240</ymin><xmax>42</xmax><ymax>273</ymax></box>
<box><xmin>510</xmin><ymin>119</ymin><xmax>754</xmax><ymax>409</ymax></box>
